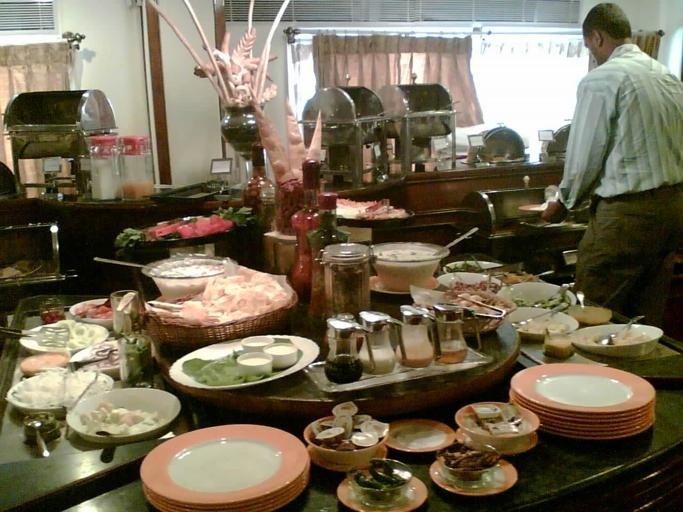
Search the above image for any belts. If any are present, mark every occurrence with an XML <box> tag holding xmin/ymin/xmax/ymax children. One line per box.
<box><xmin>604</xmin><ymin>183</ymin><xmax>683</xmax><ymax>204</ymax></box>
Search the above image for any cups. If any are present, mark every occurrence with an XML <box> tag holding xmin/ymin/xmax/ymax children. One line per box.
<box><xmin>111</xmin><ymin>290</ymin><xmax>142</xmax><ymax>334</ymax></box>
<box><xmin>39</xmin><ymin>297</ymin><xmax>64</xmax><ymax>321</ymax></box>
<box><xmin>117</xmin><ymin>335</ymin><xmax>154</xmax><ymax>388</ymax></box>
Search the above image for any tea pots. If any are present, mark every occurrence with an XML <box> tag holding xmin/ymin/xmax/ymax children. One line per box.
<box><xmin>87</xmin><ymin>136</ymin><xmax>120</xmax><ymax>202</ymax></box>
<box><xmin>112</xmin><ymin>135</ymin><xmax>154</xmax><ymax>199</ymax></box>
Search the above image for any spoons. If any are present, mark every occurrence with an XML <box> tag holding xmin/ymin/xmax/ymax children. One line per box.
<box><xmin>27</xmin><ymin>421</ymin><xmax>48</xmax><ymax>459</ymax></box>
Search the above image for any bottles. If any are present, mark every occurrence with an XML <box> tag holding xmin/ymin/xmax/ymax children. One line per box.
<box><xmin>387</xmin><ymin>121</ymin><xmax>402</xmax><ymax>158</ymax></box>
<box><xmin>242</xmin><ymin>150</ymin><xmax>507</xmax><ymax>381</ymax></box>
<box><xmin>22</xmin><ymin>411</ymin><xmax>59</xmax><ymax>443</ymax></box>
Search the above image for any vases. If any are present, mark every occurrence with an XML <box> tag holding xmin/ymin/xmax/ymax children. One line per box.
<box><xmin>220</xmin><ymin>105</ymin><xmax>263</xmax><ymax>154</ymax></box>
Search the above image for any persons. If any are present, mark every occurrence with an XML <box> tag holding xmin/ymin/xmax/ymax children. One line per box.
<box><xmin>541</xmin><ymin>2</ymin><xmax>682</xmax><ymax>327</ymax></box>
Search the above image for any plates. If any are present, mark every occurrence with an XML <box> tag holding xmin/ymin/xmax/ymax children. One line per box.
<box><xmin>67</xmin><ymin>296</ymin><xmax>112</xmax><ymax>325</ymax></box>
<box><xmin>338</xmin><ymin>473</ymin><xmax>429</xmax><ymax>512</ymax></box>
<box><xmin>518</xmin><ymin>205</ymin><xmax>547</xmax><ymax>213</ymax></box>
<box><xmin>571</xmin><ymin>323</ymin><xmax>663</xmax><ymax>358</ymax></box>
<box><xmin>67</xmin><ymin>387</ymin><xmax>180</xmax><ymax>445</ymax></box>
<box><xmin>447</xmin><ymin>426</ymin><xmax>540</xmax><ymax>453</ymax></box>
<box><xmin>6</xmin><ymin>321</ymin><xmax>124</xmax><ymax>418</ymax></box>
<box><xmin>138</xmin><ymin>215</ymin><xmax>235</xmax><ymax>248</ymax></box>
<box><xmin>497</xmin><ymin>282</ymin><xmax>581</xmax><ymax>312</ymax></box>
<box><xmin>304</xmin><ymin>444</ymin><xmax>391</xmax><ymax>474</ymax></box>
<box><xmin>381</xmin><ymin>419</ymin><xmax>458</xmax><ymax>454</ymax></box>
<box><xmin>425</xmin><ymin>455</ymin><xmax>519</xmax><ymax>496</ymax></box>
<box><xmin>510</xmin><ymin>307</ymin><xmax>579</xmax><ymax>340</ymax></box>
<box><xmin>168</xmin><ymin>334</ymin><xmax>321</xmax><ymax>390</ymax></box>
<box><xmin>140</xmin><ymin>422</ymin><xmax>312</xmax><ymax>512</ymax></box>
<box><xmin>501</xmin><ymin>362</ymin><xmax>656</xmax><ymax>442</ymax></box>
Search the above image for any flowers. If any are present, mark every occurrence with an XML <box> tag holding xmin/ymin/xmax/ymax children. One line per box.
<box><xmin>146</xmin><ymin>0</ymin><xmax>292</xmax><ymax>109</ymax></box>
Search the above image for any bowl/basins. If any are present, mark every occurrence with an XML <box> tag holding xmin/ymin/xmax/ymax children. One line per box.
<box><xmin>436</xmin><ymin>443</ymin><xmax>503</xmax><ymax>481</ymax></box>
<box><xmin>454</xmin><ymin>401</ymin><xmax>542</xmax><ymax>450</ymax></box>
<box><xmin>345</xmin><ymin>460</ymin><xmax>414</xmax><ymax>506</ymax></box>
<box><xmin>297</xmin><ymin>411</ymin><xmax>391</xmax><ymax>460</ymax></box>
<box><xmin>567</xmin><ymin>305</ymin><xmax>613</xmax><ymax>325</ymax></box>
<box><xmin>140</xmin><ymin>254</ymin><xmax>230</xmax><ymax>299</ymax></box>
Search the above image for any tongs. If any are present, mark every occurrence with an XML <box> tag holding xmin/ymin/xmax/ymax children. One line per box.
<box><xmin>0</xmin><ymin>326</ymin><xmax>75</xmax><ymax>347</ymax></box>
